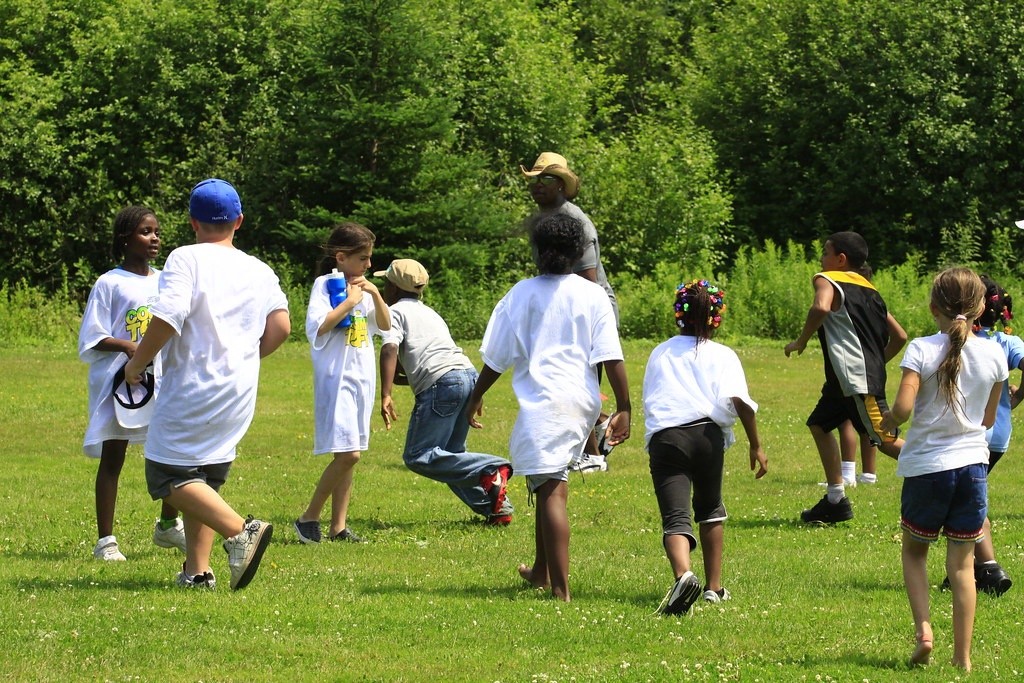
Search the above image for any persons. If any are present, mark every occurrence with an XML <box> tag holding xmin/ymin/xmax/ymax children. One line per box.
<box><xmin>941</xmin><ymin>274</ymin><xmax>1024</xmax><ymax>598</ymax></box>
<box><xmin>294</xmin><ymin>224</ymin><xmax>390</xmax><ymax>545</ymax></box>
<box><xmin>126</xmin><ymin>178</ymin><xmax>292</xmax><ymax>592</ymax></box>
<box><xmin>784</xmin><ymin>232</ymin><xmax>909</xmax><ymax>526</ymax></box>
<box><xmin>879</xmin><ymin>268</ymin><xmax>1011</xmax><ymax>678</ymax></box>
<box><xmin>521</xmin><ymin>152</ymin><xmax>620</xmax><ymax>474</ymax></box>
<box><xmin>374</xmin><ymin>260</ymin><xmax>514</xmax><ymax>527</ymax></box>
<box><xmin>466</xmin><ymin>214</ymin><xmax>632</xmax><ymax>603</ymax></box>
<box><xmin>643</xmin><ymin>280</ymin><xmax>767</xmax><ymax>618</ymax></box>
<box><xmin>79</xmin><ymin>206</ymin><xmax>187</xmax><ymax>566</ymax></box>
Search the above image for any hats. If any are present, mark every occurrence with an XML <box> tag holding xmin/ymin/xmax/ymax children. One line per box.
<box><xmin>191</xmin><ymin>177</ymin><xmax>241</xmax><ymax>222</ymax></box>
<box><xmin>373</xmin><ymin>259</ymin><xmax>429</xmax><ymax>293</ymax></box>
<box><xmin>520</xmin><ymin>151</ymin><xmax>580</xmax><ymax>200</ymax></box>
<box><xmin>113</xmin><ymin>363</ymin><xmax>155</xmax><ymax>428</ymax></box>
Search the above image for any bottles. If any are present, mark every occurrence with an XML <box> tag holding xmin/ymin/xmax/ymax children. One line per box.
<box><xmin>326</xmin><ymin>268</ymin><xmax>351</xmax><ymax>328</ymax></box>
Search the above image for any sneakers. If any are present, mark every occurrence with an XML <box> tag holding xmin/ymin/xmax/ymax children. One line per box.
<box><xmin>704</xmin><ymin>587</ymin><xmax>730</xmax><ymax>603</ymax></box>
<box><xmin>153</xmin><ymin>518</ymin><xmax>186</xmax><ymax>554</ymax></box>
<box><xmin>482</xmin><ymin>463</ymin><xmax>509</xmax><ymax>513</ymax></box>
<box><xmin>223</xmin><ymin>514</ymin><xmax>274</xmax><ymax>589</ymax></box>
<box><xmin>569</xmin><ymin>454</ymin><xmax>608</xmax><ymax>472</ymax></box>
<box><xmin>294</xmin><ymin>519</ymin><xmax>321</xmax><ymax>545</ymax></box>
<box><xmin>176</xmin><ymin>561</ymin><xmax>216</xmax><ymax>590</ymax></box>
<box><xmin>328</xmin><ymin>528</ymin><xmax>361</xmax><ymax>543</ymax></box>
<box><xmin>595</xmin><ymin>413</ymin><xmax>617</xmax><ymax>455</ymax></box>
<box><xmin>661</xmin><ymin>571</ymin><xmax>700</xmax><ymax>615</ymax></box>
<box><xmin>482</xmin><ymin>515</ymin><xmax>512</xmax><ymax>526</ymax></box>
<box><xmin>94</xmin><ymin>536</ymin><xmax>126</xmax><ymax>562</ymax></box>
<box><xmin>802</xmin><ymin>495</ymin><xmax>856</xmax><ymax>523</ymax></box>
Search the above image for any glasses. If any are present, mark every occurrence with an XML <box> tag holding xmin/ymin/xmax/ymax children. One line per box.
<box><xmin>526</xmin><ymin>173</ymin><xmax>560</xmax><ymax>185</ymax></box>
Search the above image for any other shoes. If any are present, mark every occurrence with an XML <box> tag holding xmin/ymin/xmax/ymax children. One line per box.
<box><xmin>939</xmin><ymin>564</ymin><xmax>1011</xmax><ymax>597</ymax></box>
